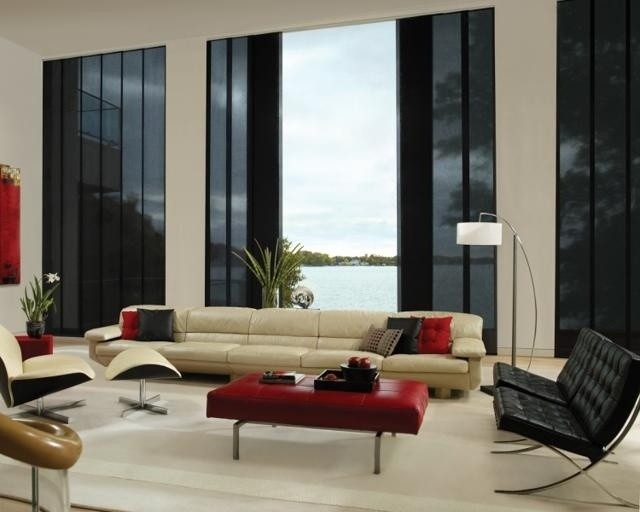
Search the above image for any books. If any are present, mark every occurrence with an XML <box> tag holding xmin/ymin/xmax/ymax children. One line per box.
<box><xmin>259</xmin><ymin>370</ymin><xmax>306</xmax><ymax>385</ymax></box>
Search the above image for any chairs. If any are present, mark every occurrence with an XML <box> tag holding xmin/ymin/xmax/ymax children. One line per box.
<box><xmin>484</xmin><ymin>344</ymin><xmax>639</xmax><ymax>510</ymax></box>
<box><xmin>488</xmin><ymin>327</ymin><xmax>620</xmax><ymax>466</ymax></box>
<box><xmin>0</xmin><ymin>322</ymin><xmax>90</xmax><ymax>426</ymax></box>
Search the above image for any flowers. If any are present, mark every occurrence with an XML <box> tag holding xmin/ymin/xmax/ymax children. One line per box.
<box><xmin>16</xmin><ymin>266</ymin><xmax>62</xmax><ymax>323</ymax></box>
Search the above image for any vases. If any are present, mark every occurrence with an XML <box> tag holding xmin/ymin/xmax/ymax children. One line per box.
<box><xmin>26</xmin><ymin>320</ymin><xmax>47</xmax><ymax>340</ymax></box>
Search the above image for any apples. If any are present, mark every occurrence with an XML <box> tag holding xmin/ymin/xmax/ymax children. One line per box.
<box><xmin>349</xmin><ymin>357</ymin><xmax>371</xmax><ymax>367</ymax></box>
<box><xmin>324</xmin><ymin>374</ymin><xmax>335</xmax><ymax>381</ymax></box>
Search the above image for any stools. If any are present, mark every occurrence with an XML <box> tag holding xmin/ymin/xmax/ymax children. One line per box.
<box><xmin>0</xmin><ymin>412</ymin><xmax>82</xmax><ymax>511</ymax></box>
<box><xmin>99</xmin><ymin>345</ymin><xmax>182</xmax><ymax>420</ymax></box>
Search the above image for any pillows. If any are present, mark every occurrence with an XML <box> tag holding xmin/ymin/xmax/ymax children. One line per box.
<box><xmin>386</xmin><ymin>316</ymin><xmax>424</xmax><ymax>354</ymax></box>
<box><xmin>359</xmin><ymin>323</ymin><xmax>405</xmax><ymax>358</ymax></box>
<box><xmin>134</xmin><ymin>304</ymin><xmax>176</xmax><ymax>343</ymax></box>
<box><xmin>416</xmin><ymin>316</ymin><xmax>453</xmax><ymax>354</ymax></box>
<box><xmin>119</xmin><ymin>311</ymin><xmax>140</xmax><ymax>342</ymax></box>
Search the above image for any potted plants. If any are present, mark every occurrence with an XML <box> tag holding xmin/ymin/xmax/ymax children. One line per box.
<box><xmin>229</xmin><ymin>234</ymin><xmax>308</xmax><ymax>308</ymax></box>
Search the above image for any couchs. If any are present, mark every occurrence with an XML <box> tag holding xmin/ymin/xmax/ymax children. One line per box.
<box><xmin>83</xmin><ymin>303</ymin><xmax>483</xmax><ymax>403</ymax></box>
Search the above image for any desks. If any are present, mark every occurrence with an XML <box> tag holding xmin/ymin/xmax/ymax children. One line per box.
<box><xmin>15</xmin><ymin>334</ymin><xmax>55</xmax><ymax>362</ymax></box>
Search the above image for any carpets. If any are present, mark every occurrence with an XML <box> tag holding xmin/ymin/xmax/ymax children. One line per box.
<box><xmin>0</xmin><ymin>343</ymin><xmax>639</xmax><ymax>510</ymax></box>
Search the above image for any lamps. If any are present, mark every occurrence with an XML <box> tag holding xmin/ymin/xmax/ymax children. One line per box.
<box><xmin>454</xmin><ymin>209</ymin><xmax>541</xmax><ymax>396</ymax></box>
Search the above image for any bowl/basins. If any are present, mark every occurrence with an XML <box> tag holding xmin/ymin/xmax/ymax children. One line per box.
<box><xmin>340</xmin><ymin>363</ymin><xmax>378</xmax><ymax>380</ymax></box>
